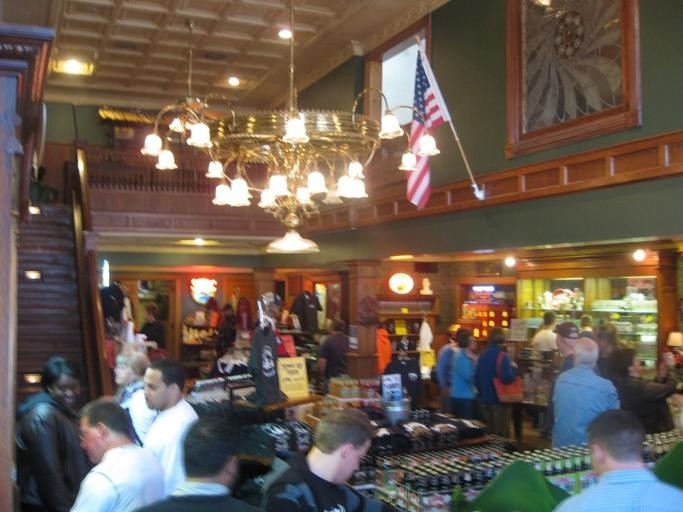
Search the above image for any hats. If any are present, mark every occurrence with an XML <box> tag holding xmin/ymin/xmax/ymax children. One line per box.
<box><xmin>553</xmin><ymin>322</ymin><xmax>579</xmax><ymax>339</ymax></box>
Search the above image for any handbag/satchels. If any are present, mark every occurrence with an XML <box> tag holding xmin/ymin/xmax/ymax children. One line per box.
<box><xmin>493</xmin><ymin>351</ymin><xmax>522</xmax><ymax>404</ymax></box>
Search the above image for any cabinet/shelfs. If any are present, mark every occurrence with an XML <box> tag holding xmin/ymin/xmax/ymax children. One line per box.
<box><xmin>515</xmin><ymin>256</ymin><xmax>586</xmax><ymax>344</ymax></box>
<box><xmin>586</xmin><ymin>249</ymin><xmax>678</xmax><ymax>381</ymax></box>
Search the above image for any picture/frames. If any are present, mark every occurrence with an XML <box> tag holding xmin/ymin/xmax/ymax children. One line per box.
<box><xmin>505</xmin><ymin>0</ymin><xmax>642</xmax><ymax>158</ymax></box>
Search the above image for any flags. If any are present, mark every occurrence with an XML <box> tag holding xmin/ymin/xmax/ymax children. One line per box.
<box><xmin>403</xmin><ymin>43</ymin><xmax>451</xmax><ymax>209</ymax></box>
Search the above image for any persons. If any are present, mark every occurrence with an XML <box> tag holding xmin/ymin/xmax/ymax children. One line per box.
<box><xmin>263</xmin><ymin>407</ymin><xmax>389</xmax><ymax>511</ymax></box>
<box><xmin>213</xmin><ymin>304</ymin><xmax>236</xmax><ymax>344</ymax></box>
<box><xmin>11</xmin><ymin>345</ymin><xmax>258</xmax><ymax>512</ymax></box>
<box><xmin>136</xmin><ymin>303</ymin><xmax>168</xmax><ymax>359</ymax></box>
<box><xmin>552</xmin><ymin>408</ymin><xmax>683</xmax><ymax>512</ymax></box>
<box><xmin>316</xmin><ymin>319</ymin><xmax>350</xmax><ymax>377</ymax></box>
<box><xmin>436</xmin><ymin>327</ymin><xmax>520</xmax><ymax>438</ymax></box>
<box><xmin>529</xmin><ymin>313</ymin><xmax>683</xmax><ymax>449</ymax></box>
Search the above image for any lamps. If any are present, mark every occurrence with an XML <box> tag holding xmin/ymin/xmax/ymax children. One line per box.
<box><xmin>164</xmin><ymin>20</ymin><xmax>215</xmax><ymax>149</ymax></box>
<box><xmin>137</xmin><ymin>0</ymin><xmax>442</xmax><ymax>256</ymax></box>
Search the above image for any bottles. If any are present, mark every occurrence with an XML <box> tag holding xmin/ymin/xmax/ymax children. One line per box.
<box><xmin>304</xmin><ymin>371</ymin><xmax>383</xmax><ymax>442</ymax></box>
<box><xmin>353</xmin><ymin>425</ymin><xmax>681</xmax><ymax>511</ymax></box>
<box><xmin>180</xmin><ymin>309</ymin><xmax>220</xmax><ymax>344</ymax></box>
<box><xmin>376</xmin><ymin>299</ymin><xmax>432</xmax><ymax>313</ymax></box>
